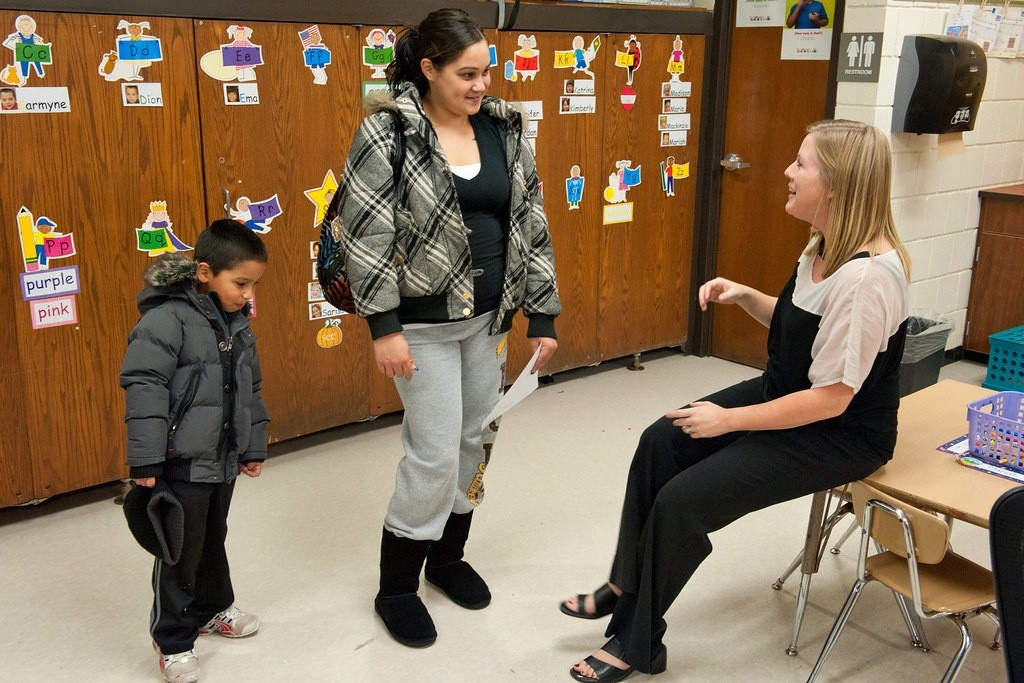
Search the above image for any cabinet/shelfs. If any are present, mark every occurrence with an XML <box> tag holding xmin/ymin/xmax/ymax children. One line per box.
<box><xmin>961</xmin><ymin>184</ymin><xmax>1024</xmax><ymax>359</ymax></box>
<box><xmin>0</xmin><ymin>0</ymin><xmax>719</xmax><ymax>515</ymax></box>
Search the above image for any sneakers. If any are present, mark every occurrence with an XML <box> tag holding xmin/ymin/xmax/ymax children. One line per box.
<box><xmin>151</xmin><ymin>638</ymin><xmax>203</xmax><ymax>683</ymax></box>
<box><xmin>197</xmin><ymin>603</ymin><xmax>260</xmax><ymax>639</ymax></box>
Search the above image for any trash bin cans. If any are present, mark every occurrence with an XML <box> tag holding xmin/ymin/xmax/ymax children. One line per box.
<box><xmin>898</xmin><ymin>316</ymin><xmax>953</xmax><ymax>399</ymax></box>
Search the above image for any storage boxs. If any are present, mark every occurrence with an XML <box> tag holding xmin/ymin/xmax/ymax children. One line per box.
<box><xmin>965</xmin><ymin>325</ymin><xmax>1024</xmax><ymax>475</ymax></box>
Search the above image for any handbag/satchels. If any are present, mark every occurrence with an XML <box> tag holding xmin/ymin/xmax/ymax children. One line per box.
<box><xmin>315</xmin><ymin>107</ymin><xmax>408</xmax><ymax>314</ymax></box>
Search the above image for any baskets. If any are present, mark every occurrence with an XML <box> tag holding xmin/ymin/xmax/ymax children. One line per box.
<box><xmin>964</xmin><ymin>390</ymin><xmax>1024</xmax><ymax>472</ymax></box>
<box><xmin>980</xmin><ymin>324</ymin><xmax>1024</xmax><ymax>393</ymax></box>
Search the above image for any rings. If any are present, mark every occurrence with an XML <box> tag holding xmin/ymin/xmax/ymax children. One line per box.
<box><xmin>687</xmin><ymin>426</ymin><xmax>692</xmax><ymax>434</ymax></box>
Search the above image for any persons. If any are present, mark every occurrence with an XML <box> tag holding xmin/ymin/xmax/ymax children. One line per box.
<box><xmin>118</xmin><ymin>218</ymin><xmax>272</xmax><ymax>683</ymax></box>
<box><xmin>341</xmin><ymin>7</ymin><xmax>562</xmax><ymax>649</ymax></box>
<box><xmin>558</xmin><ymin>119</ymin><xmax>911</xmax><ymax>683</ymax></box>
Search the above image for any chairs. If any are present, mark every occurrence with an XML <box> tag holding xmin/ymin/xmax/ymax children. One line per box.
<box><xmin>771</xmin><ymin>479</ymin><xmax>1024</xmax><ymax>683</ymax></box>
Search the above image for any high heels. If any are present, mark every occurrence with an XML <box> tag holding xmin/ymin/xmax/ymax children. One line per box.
<box><xmin>570</xmin><ymin>634</ymin><xmax>668</xmax><ymax>683</ymax></box>
<box><xmin>558</xmin><ymin>579</ymin><xmax>621</xmax><ymax>620</ymax></box>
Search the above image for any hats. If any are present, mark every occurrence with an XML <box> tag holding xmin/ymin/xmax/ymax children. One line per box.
<box><xmin>112</xmin><ymin>476</ymin><xmax>186</xmax><ymax>565</ymax></box>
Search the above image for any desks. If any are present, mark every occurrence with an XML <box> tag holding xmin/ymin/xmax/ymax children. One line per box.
<box><xmin>786</xmin><ymin>377</ymin><xmax>1024</xmax><ymax>656</ymax></box>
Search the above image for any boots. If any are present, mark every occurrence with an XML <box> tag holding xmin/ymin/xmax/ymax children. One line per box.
<box><xmin>374</xmin><ymin>522</ymin><xmax>438</xmax><ymax>650</ymax></box>
<box><xmin>422</xmin><ymin>509</ymin><xmax>493</xmax><ymax>611</ymax></box>
